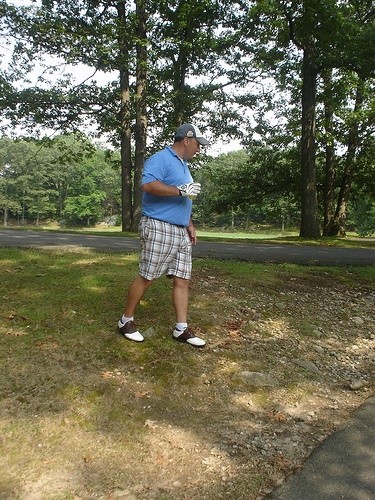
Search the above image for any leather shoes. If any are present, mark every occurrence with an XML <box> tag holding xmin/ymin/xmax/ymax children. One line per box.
<box><xmin>172</xmin><ymin>327</ymin><xmax>206</xmax><ymax>347</ymax></box>
<box><xmin>117</xmin><ymin>320</ymin><xmax>144</xmax><ymax>342</ymax></box>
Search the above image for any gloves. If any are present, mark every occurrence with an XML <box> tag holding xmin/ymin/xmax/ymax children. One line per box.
<box><xmin>176</xmin><ymin>182</ymin><xmax>201</xmax><ymax>200</ymax></box>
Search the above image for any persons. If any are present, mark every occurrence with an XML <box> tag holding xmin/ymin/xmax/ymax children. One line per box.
<box><xmin>117</xmin><ymin>123</ymin><xmax>207</xmax><ymax>348</ymax></box>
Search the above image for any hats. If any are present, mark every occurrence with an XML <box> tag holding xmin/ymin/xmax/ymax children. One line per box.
<box><xmin>174</xmin><ymin>124</ymin><xmax>210</xmax><ymax>146</ymax></box>
